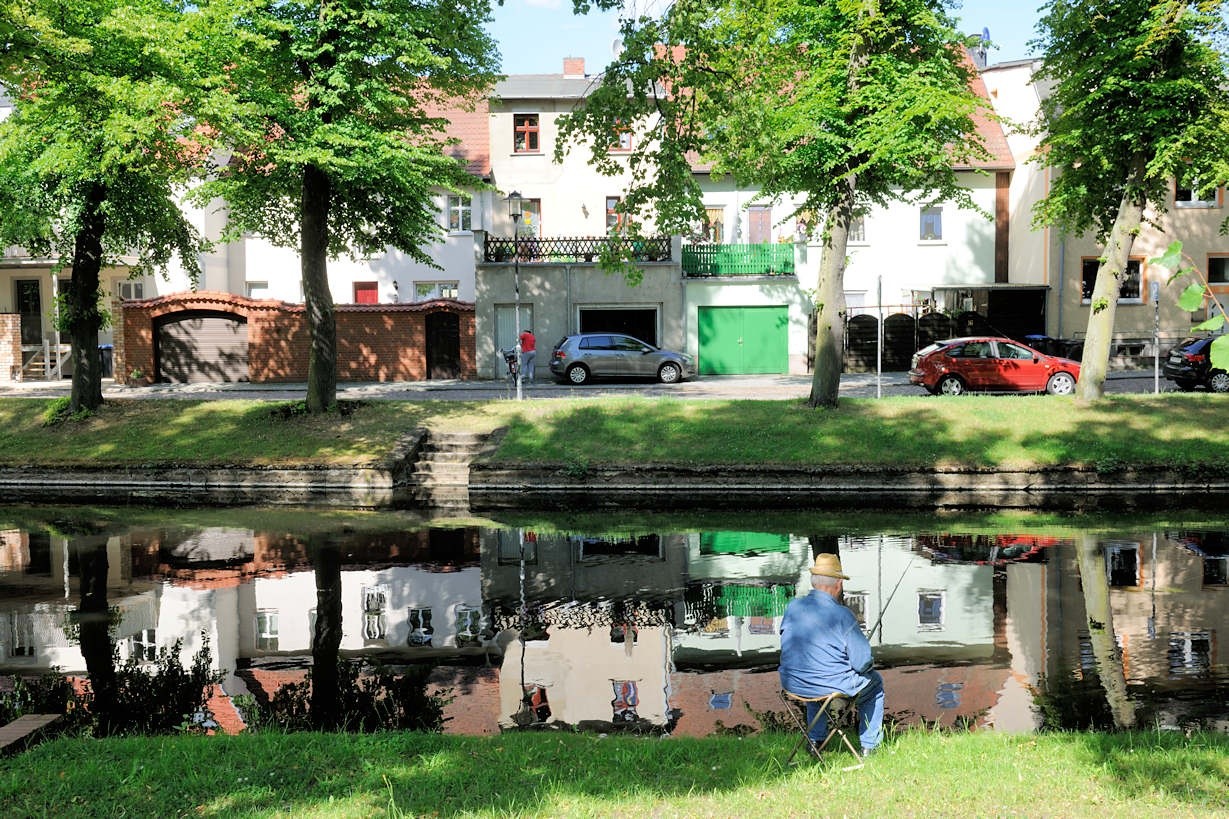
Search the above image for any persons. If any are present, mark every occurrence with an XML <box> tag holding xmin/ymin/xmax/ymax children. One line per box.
<box><xmin>519</xmin><ymin>330</ymin><xmax>536</xmax><ymax>383</ymax></box>
<box><xmin>778</xmin><ymin>553</ymin><xmax>885</xmax><ymax>758</ymax></box>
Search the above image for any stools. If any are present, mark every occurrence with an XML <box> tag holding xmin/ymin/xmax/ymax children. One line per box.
<box><xmin>780</xmin><ymin>690</ymin><xmax>864</xmax><ymax>772</ymax></box>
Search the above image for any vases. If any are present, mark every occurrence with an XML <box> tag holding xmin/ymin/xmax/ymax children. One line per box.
<box><xmin>584</xmin><ymin>253</ymin><xmax>592</xmax><ymax>262</ymax></box>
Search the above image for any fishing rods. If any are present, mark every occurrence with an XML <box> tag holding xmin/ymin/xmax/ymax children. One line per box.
<box><xmin>867</xmin><ymin>544</ymin><xmax>926</xmax><ymax>643</ymax></box>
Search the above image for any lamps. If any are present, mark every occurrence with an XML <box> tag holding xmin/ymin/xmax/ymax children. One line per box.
<box><xmin>393</xmin><ymin>280</ymin><xmax>398</xmax><ymax>290</ymax></box>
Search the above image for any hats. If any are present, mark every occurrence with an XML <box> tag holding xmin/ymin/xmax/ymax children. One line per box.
<box><xmin>808</xmin><ymin>552</ymin><xmax>850</xmax><ymax>581</ymax></box>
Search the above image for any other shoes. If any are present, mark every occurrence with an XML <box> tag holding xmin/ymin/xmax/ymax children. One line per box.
<box><xmin>861</xmin><ymin>747</ymin><xmax>872</xmax><ymax>758</ymax></box>
<box><xmin>807</xmin><ymin>741</ymin><xmax>823</xmax><ymax>756</ymax></box>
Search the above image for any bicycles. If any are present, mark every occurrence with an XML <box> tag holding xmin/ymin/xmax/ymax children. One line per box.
<box><xmin>500</xmin><ymin>345</ymin><xmax>518</xmax><ymax>387</ymax></box>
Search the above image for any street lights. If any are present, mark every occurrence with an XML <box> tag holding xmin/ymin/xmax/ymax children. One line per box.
<box><xmin>506</xmin><ymin>188</ymin><xmax>523</xmax><ymax>404</ymax></box>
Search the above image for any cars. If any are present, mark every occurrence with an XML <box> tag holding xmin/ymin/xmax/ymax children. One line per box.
<box><xmin>1162</xmin><ymin>334</ymin><xmax>1229</xmax><ymax>393</ymax></box>
<box><xmin>910</xmin><ymin>338</ymin><xmax>1082</xmax><ymax>397</ymax></box>
<box><xmin>549</xmin><ymin>332</ymin><xmax>697</xmax><ymax>385</ymax></box>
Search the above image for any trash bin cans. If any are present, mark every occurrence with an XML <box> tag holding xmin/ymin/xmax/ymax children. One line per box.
<box><xmin>1048</xmin><ymin>337</ymin><xmax>1085</xmax><ymax>362</ymax></box>
<box><xmin>132</xmin><ymin>544</ymin><xmax>142</xmax><ymax>566</ymax></box>
<box><xmin>1014</xmin><ymin>548</ymin><xmax>1049</xmax><ymax>565</ymax></box>
<box><xmin>1025</xmin><ymin>335</ymin><xmax>1048</xmax><ymax>352</ymax></box>
<box><xmin>98</xmin><ymin>345</ymin><xmax>113</xmax><ymax>378</ymax></box>
